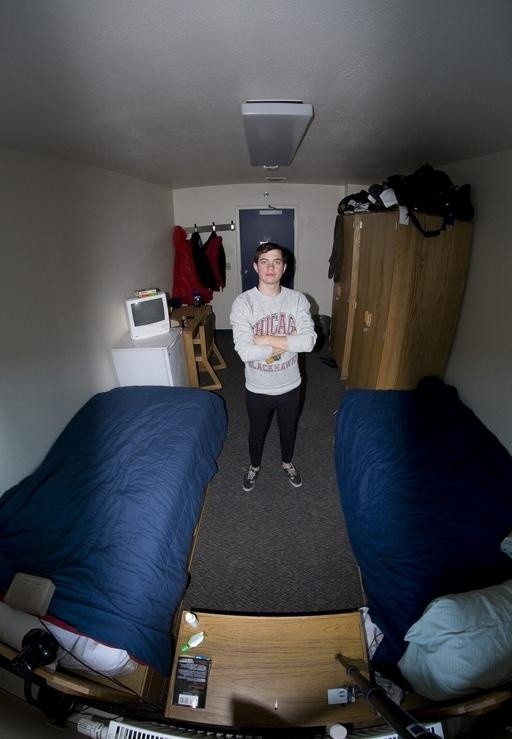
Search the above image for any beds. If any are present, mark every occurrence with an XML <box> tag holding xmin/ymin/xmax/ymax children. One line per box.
<box><xmin>0</xmin><ymin>382</ymin><xmax>227</xmax><ymax>711</ymax></box>
<box><xmin>332</xmin><ymin>375</ymin><xmax>511</xmax><ymax>720</ymax></box>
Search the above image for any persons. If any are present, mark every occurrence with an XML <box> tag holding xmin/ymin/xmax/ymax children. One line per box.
<box><xmin>230</xmin><ymin>243</ymin><xmax>317</xmax><ymax>491</ymax></box>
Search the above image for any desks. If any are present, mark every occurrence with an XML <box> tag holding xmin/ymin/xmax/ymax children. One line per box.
<box><xmin>170</xmin><ymin>304</ymin><xmax>212</xmax><ymax>390</ymax></box>
<box><xmin>158</xmin><ymin>608</ymin><xmax>377</xmax><ymax>726</ymax></box>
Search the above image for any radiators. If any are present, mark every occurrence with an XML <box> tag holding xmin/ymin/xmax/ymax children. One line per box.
<box><xmin>78</xmin><ymin>703</ymin><xmax>398</xmax><ymax>738</ymax></box>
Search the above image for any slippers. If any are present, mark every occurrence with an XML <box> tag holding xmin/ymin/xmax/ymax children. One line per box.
<box><xmin>319</xmin><ymin>356</ymin><xmax>338</xmax><ymax>368</ymax></box>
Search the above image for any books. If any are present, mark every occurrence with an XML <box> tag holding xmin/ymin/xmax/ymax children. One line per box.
<box><xmin>171</xmin><ymin>655</ymin><xmax>213</xmax><ymax>710</ymax></box>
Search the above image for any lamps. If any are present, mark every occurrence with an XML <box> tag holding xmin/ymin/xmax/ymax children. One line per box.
<box><xmin>240</xmin><ymin>96</ymin><xmax>314</xmax><ymax>168</ymax></box>
<box><xmin>11</xmin><ymin>624</ymin><xmax>77</xmax><ymax>727</ymax></box>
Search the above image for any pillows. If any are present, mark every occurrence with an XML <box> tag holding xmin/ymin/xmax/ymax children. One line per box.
<box><xmin>397</xmin><ymin>581</ymin><xmax>512</xmax><ymax>699</ymax></box>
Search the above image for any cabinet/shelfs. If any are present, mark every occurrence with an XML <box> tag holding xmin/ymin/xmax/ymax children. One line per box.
<box><xmin>328</xmin><ymin>211</ymin><xmax>474</xmax><ymax>391</ymax></box>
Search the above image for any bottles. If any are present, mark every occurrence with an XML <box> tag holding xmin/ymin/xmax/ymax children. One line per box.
<box><xmin>185</xmin><ymin>613</ymin><xmax>200</xmax><ymax>628</ymax></box>
<box><xmin>193</xmin><ymin>289</ymin><xmax>201</xmax><ymax>307</ymax></box>
<box><xmin>181</xmin><ymin>632</ymin><xmax>208</xmax><ymax>652</ymax></box>
<box><xmin>181</xmin><ymin>316</ymin><xmax>187</xmax><ymax>328</ymax></box>
<box><xmin>326</xmin><ymin>722</ymin><xmax>347</xmax><ymax>738</ymax></box>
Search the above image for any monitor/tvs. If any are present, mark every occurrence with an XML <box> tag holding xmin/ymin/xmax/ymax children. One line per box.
<box><xmin>124</xmin><ymin>291</ymin><xmax>170</xmax><ymax>340</ymax></box>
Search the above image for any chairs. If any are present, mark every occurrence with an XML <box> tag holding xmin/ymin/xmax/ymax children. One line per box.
<box><xmin>192</xmin><ymin>312</ymin><xmax>226</xmax><ymax>390</ymax></box>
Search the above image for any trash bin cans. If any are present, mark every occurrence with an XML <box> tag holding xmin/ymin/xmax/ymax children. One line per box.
<box><xmin>310</xmin><ymin>315</ymin><xmax>330</xmax><ymax>353</ymax></box>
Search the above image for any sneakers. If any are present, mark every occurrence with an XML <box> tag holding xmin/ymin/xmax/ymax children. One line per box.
<box><xmin>242</xmin><ymin>464</ymin><xmax>261</xmax><ymax>491</ymax></box>
<box><xmin>280</xmin><ymin>462</ymin><xmax>302</xmax><ymax>488</ymax></box>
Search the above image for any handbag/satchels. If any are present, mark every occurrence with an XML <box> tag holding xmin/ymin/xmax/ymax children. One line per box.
<box><xmin>379</xmin><ymin>163</ymin><xmax>475</xmax><ymax>221</ymax></box>
